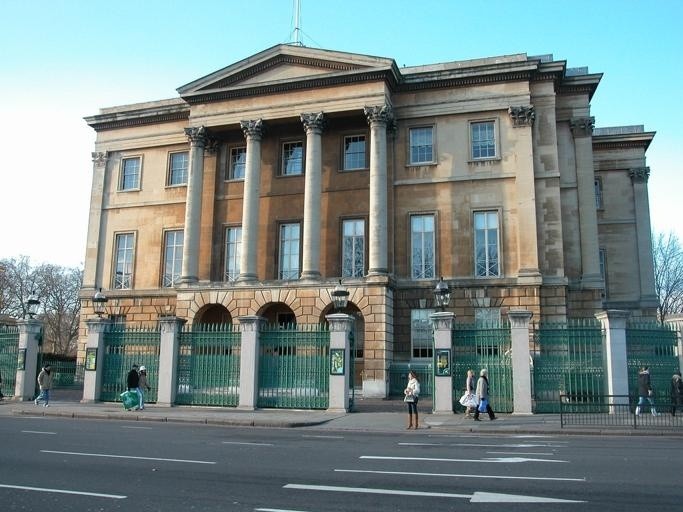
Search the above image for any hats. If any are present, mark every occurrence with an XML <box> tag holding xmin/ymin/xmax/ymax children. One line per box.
<box><xmin>139</xmin><ymin>365</ymin><xmax>146</xmax><ymax>372</ymax></box>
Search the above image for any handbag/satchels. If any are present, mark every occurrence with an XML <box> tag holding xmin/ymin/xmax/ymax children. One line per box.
<box><xmin>404</xmin><ymin>394</ymin><xmax>415</xmax><ymax>403</ymax></box>
<box><xmin>478</xmin><ymin>399</ymin><xmax>490</xmax><ymax>413</ymax></box>
<box><xmin>120</xmin><ymin>390</ymin><xmax>138</xmax><ymax>411</ymax></box>
<box><xmin>458</xmin><ymin>392</ymin><xmax>479</xmax><ymax>408</ymax></box>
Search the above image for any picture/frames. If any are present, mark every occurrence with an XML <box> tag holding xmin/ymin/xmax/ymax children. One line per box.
<box><xmin>435</xmin><ymin>348</ymin><xmax>451</xmax><ymax>376</ymax></box>
<box><xmin>85</xmin><ymin>348</ymin><xmax>97</xmax><ymax>370</ymax></box>
<box><xmin>17</xmin><ymin>348</ymin><xmax>26</xmax><ymax>370</ymax></box>
<box><xmin>331</xmin><ymin>349</ymin><xmax>344</xmax><ymax>375</ymax></box>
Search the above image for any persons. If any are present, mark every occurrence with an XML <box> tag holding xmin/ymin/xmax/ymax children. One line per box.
<box><xmin>404</xmin><ymin>371</ymin><xmax>421</xmax><ymax>430</ymax></box>
<box><xmin>671</xmin><ymin>371</ymin><xmax>683</xmax><ymax>416</ymax></box>
<box><xmin>135</xmin><ymin>366</ymin><xmax>151</xmax><ymax>410</ymax></box>
<box><xmin>635</xmin><ymin>367</ymin><xmax>661</xmax><ymax>417</ymax></box>
<box><xmin>35</xmin><ymin>365</ymin><xmax>53</xmax><ymax>407</ymax></box>
<box><xmin>473</xmin><ymin>369</ymin><xmax>498</xmax><ymax>421</ymax></box>
<box><xmin>127</xmin><ymin>364</ymin><xmax>143</xmax><ymax>411</ymax></box>
<box><xmin>459</xmin><ymin>370</ymin><xmax>476</xmax><ymax>419</ymax></box>
